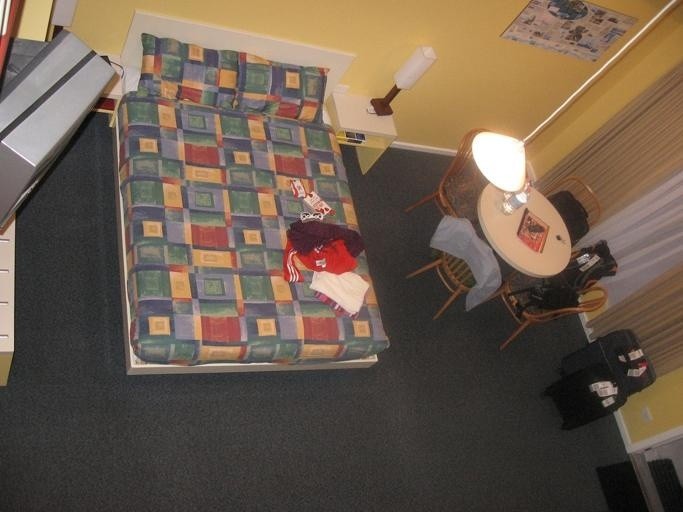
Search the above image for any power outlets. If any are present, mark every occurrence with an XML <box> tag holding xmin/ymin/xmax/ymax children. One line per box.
<box><xmin>642</xmin><ymin>409</ymin><xmax>652</xmax><ymax>422</ymax></box>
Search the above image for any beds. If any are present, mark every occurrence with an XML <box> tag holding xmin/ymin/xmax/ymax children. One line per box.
<box><xmin>109</xmin><ymin>9</ymin><xmax>389</xmax><ymax>374</ymax></box>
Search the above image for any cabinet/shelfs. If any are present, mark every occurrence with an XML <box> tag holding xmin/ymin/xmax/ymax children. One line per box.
<box><xmin>0</xmin><ymin>213</ymin><xmax>15</xmax><ymax>386</ymax></box>
<box><xmin>0</xmin><ymin>30</ymin><xmax>116</xmax><ymax>234</ymax></box>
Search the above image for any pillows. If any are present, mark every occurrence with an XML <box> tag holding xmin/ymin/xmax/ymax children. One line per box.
<box><xmin>138</xmin><ymin>33</ymin><xmax>239</xmax><ymax>111</ymax></box>
<box><xmin>239</xmin><ymin>52</ymin><xmax>329</xmax><ymax>125</ymax></box>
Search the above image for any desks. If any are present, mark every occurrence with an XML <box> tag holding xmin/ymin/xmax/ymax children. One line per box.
<box><xmin>478</xmin><ymin>183</ymin><xmax>572</xmax><ymax>277</ymax></box>
<box><xmin>540</xmin><ymin>330</ymin><xmax>656</xmax><ymax>431</ymax></box>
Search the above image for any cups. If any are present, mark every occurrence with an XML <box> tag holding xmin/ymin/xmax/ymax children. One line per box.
<box><xmin>501</xmin><ymin>191</ymin><xmax>529</xmax><ymax>216</ymax></box>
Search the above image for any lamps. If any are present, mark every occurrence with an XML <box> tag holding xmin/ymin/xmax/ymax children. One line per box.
<box><xmin>471</xmin><ymin>1</ymin><xmax>683</xmax><ymax>193</ymax></box>
<box><xmin>370</xmin><ymin>47</ymin><xmax>438</xmax><ymax>116</ymax></box>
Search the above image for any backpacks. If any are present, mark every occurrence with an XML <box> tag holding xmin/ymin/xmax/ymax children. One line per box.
<box><xmin>559</xmin><ymin>240</ymin><xmax>617</xmax><ymax>295</ymax></box>
<box><xmin>508</xmin><ymin>275</ymin><xmax>579</xmax><ymax>318</ymax></box>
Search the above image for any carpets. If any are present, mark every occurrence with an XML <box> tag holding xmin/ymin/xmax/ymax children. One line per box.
<box><xmin>596</xmin><ymin>458</ymin><xmax>683</xmax><ymax>512</ymax></box>
<box><xmin>0</xmin><ymin>109</ymin><xmax>631</xmax><ymax>512</ymax></box>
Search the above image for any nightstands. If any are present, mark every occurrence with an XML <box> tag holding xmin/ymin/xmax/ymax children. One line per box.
<box><xmin>326</xmin><ymin>93</ymin><xmax>397</xmax><ymax>174</ymax></box>
<box><xmin>91</xmin><ymin>52</ymin><xmax>123</xmax><ymax>128</ymax></box>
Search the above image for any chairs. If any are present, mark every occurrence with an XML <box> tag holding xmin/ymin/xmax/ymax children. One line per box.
<box><xmin>406</xmin><ymin>216</ymin><xmax>502</xmax><ymax>321</ymax></box>
<box><xmin>570</xmin><ymin>240</ymin><xmax>617</xmax><ymax>289</ymax></box>
<box><xmin>483</xmin><ymin>270</ymin><xmax>608</xmax><ymax>351</ymax></box>
<box><xmin>543</xmin><ymin>176</ymin><xmax>601</xmax><ymax>249</ymax></box>
<box><xmin>404</xmin><ymin>129</ymin><xmax>493</xmax><ymax>224</ymax></box>
